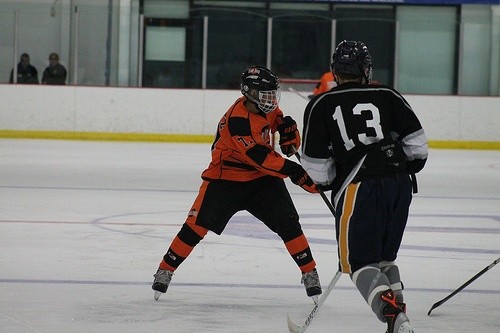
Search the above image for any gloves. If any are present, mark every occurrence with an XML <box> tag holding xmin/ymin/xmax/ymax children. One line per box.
<box><xmin>277</xmin><ymin>116</ymin><xmax>301</xmax><ymax>158</ymax></box>
<box><xmin>288</xmin><ymin>162</ymin><xmax>319</xmax><ymax>194</ymax></box>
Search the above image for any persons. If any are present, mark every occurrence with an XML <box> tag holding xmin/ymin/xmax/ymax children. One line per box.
<box><xmin>10</xmin><ymin>52</ymin><xmax>68</xmax><ymax>86</ymax></box>
<box><xmin>298</xmin><ymin>39</ymin><xmax>428</xmax><ymax>333</ymax></box>
<box><xmin>152</xmin><ymin>65</ymin><xmax>322</xmax><ymax>297</ymax></box>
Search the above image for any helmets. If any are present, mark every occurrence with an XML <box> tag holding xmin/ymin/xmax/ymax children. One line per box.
<box><xmin>330</xmin><ymin>39</ymin><xmax>374</xmax><ymax>85</ymax></box>
<box><xmin>240</xmin><ymin>65</ymin><xmax>281</xmax><ymax>115</ymax></box>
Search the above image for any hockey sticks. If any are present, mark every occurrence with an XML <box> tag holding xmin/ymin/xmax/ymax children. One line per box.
<box><xmin>427</xmin><ymin>256</ymin><xmax>500</xmax><ymax>316</ymax></box>
<box><xmin>284</xmin><ymin>270</ymin><xmax>343</xmax><ymax>333</ymax></box>
<box><xmin>292</xmin><ymin>145</ymin><xmax>336</xmax><ymax>218</ymax></box>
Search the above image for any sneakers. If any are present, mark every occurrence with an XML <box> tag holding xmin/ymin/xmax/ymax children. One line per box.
<box><xmin>151</xmin><ymin>266</ymin><xmax>174</xmax><ymax>302</ymax></box>
<box><xmin>300</xmin><ymin>267</ymin><xmax>323</xmax><ymax>307</ymax></box>
<box><xmin>378</xmin><ymin>288</ymin><xmax>415</xmax><ymax>333</ymax></box>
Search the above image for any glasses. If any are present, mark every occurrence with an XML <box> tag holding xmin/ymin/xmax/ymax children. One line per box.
<box><xmin>50</xmin><ymin>57</ymin><xmax>57</xmax><ymax>61</ymax></box>
